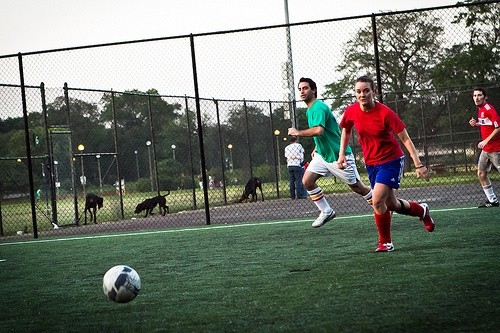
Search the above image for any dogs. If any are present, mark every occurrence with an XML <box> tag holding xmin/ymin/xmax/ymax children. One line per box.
<box><xmin>134</xmin><ymin>190</ymin><xmax>170</xmax><ymax>218</ymax></box>
<box><xmin>240</xmin><ymin>176</ymin><xmax>264</xmax><ymax>202</ymax></box>
<box><xmin>84</xmin><ymin>193</ymin><xmax>103</xmax><ymax>225</ymax></box>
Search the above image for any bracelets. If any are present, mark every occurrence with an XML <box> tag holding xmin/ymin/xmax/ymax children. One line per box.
<box><xmin>416</xmin><ymin>165</ymin><xmax>424</xmax><ymax>170</ymax></box>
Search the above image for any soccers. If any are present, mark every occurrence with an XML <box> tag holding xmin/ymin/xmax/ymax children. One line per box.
<box><xmin>102</xmin><ymin>264</ymin><xmax>142</xmax><ymax>304</ymax></box>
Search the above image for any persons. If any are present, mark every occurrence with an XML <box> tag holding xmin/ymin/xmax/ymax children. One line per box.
<box><xmin>285</xmin><ymin>136</ymin><xmax>307</xmax><ymax>199</ymax></box>
<box><xmin>337</xmin><ymin>77</ymin><xmax>436</xmax><ymax>252</ymax></box>
<box><xmin>469</xmin><ymin>88</ymin><xmax>500</xmax><ymax>208</ymax></box>
<box><xmin>288</xmin><ymin>77</ymin><xmax>393</xmax><ymax>228</ymax></box>
<box><xmin>35</xmin><ymin>171</ymin><xmax>238</xmax><ymax>209</ymax></box>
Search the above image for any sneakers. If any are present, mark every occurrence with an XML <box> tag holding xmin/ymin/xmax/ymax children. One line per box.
<box><xmin>312</xmin><ymin>207</ymin><xmax>335</xmax><ymax>228</ymax></box>
<box><xmin>374</xmin><ymin>242</ymin><xmax>394</xmax><ymax>252</ymax></box>
<box><xmin>419</xmin><ymin>202</ymin><xmax>435</xmax><ymax>231</ymax></box>
<box><xmin>478</xmin><ymin>199</ymin><xmax>499</xmax><ymax>207</ymax></box>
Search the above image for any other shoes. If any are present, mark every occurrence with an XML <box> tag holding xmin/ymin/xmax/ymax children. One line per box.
<box><xmin>292</xmin><ymin>196</ymin><xmax>295</xmax><ymax>199</ymax></box>
<box><xmin>297</xmin><ymin>195</ymin><xmax>307</xmax><ymax>198</ymax></box>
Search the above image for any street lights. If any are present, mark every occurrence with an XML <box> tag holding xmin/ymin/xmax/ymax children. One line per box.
<box><xmin>134</xmin><ymin>150</ymin><xmax>140</xmax><ymax>180</ymax></box>
<box><xmin>274</xmin><ymin>130</ymin><xmax>282</xmax><ymax>192</ymax></box>
<box><xmin>171</xmin><ymin>145</ymin><xmax>176</xmax><ymax>160</ymax></box>
<box><xmin>228</xmin><ymin>144</ymin><xmax>233</xmax><ymax>174</ymax></box>
<box><xmin>78</xmin><ymin>144</ymin><xmax>86</xmax><ymax>202</ymax></box>
<box><xmin>96</xmin><ymin>154</ymin><xmax>103</xmax><ymax>197</ymax></box>
<box><xmin>54</xmin><ymin>161</ymin><xmax>59</xmax><ymax>199</ymax></box>
<box><xmin>146</xmin><ymin>141</ymin><xmax>154</xmax><ymax>192</ymax></box>
<box><xmin>17</xmin><ymin>158</ymin><xmax>23</xmax><ymax>197</ymax></box>
